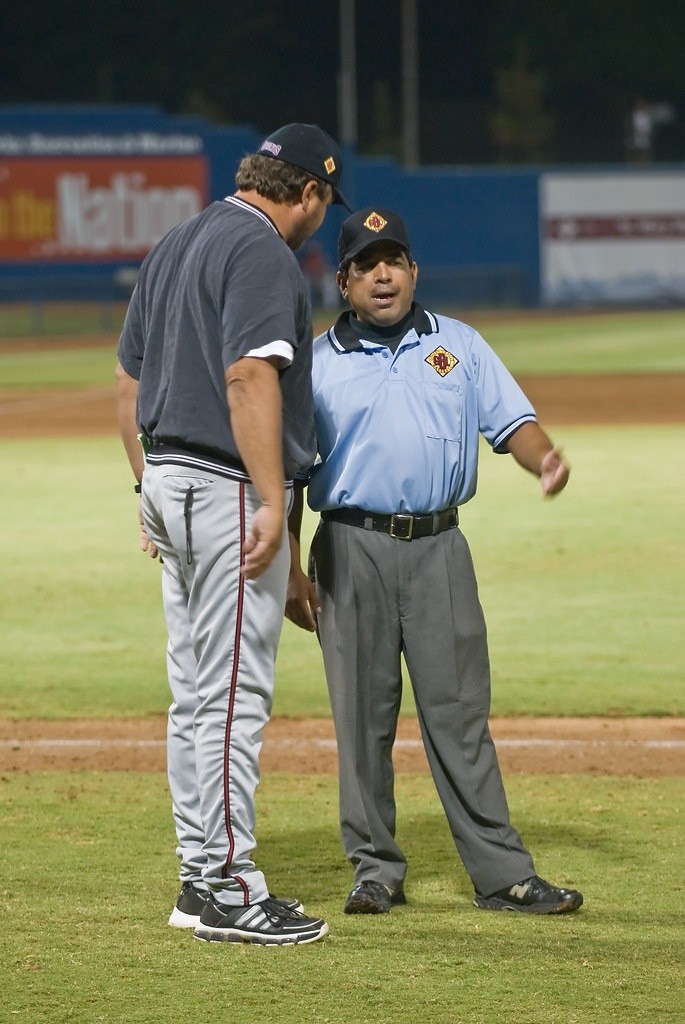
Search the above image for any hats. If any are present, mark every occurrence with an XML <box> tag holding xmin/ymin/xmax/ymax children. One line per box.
<box><xmin>338</xmin><ymin>207</ymin><xmax>409</xmax><ymax>263</ymax></box>
<box><xmin>256</xmin><ymin>123</ymin><xmax>354</xmax><ymax>215</ymax></box>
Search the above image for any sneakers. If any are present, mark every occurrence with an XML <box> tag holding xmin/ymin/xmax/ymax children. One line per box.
<box><xmin>473</xmin><ymin>875</ymin><xmax>583</xmax><ymax>915</ymax></box>
<box><xmin>344</xmin><ymin>879</ymin><xmax>406</xmax><ymax>914</ymax></box>
<box><xmin>168</xmin><ymin>881</ymin><xmax>305</xmax><ymax>928</ymax></box>
<box><xmin>193</xmin><ymin>891</ymin><xmax>329</xmax><ymax>946</ymax></box>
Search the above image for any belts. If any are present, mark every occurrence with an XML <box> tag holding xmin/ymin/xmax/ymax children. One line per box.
<box><xmin>321</xmin><ymin>507</ymin><xmax>456</xmax><ymax>541</ymax></box>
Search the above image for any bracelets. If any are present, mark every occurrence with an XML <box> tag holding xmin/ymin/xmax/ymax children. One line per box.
<box><xmin>133</xmin><ymin>482</ymin><xmax>143</xmax><ymax>494</ymax></box>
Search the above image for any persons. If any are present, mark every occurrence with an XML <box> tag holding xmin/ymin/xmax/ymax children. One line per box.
<box><xmin>279</xmin><ymin>207</ymin><xmax>584</xmax><ymax>916</ymax></box>
<box><xmin>113</xmin><ymin>122</ymin><xmax>355</xmax><ymax>947</ymax></box>
